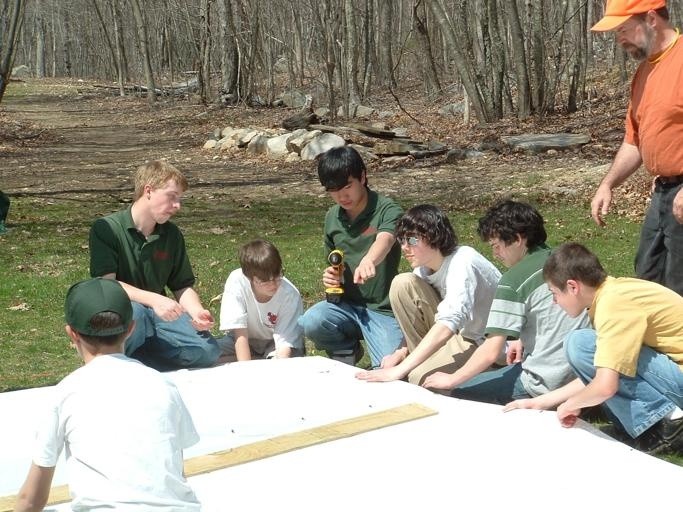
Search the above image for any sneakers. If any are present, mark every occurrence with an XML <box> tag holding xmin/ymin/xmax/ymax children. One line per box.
<box><xmin>333</xmin><ymin>343</ymin><xmax>365</xmax><ymax>365</ymax></box>
<box><xmin>623</xmin><ymin>416</ymin><xmax>682</xmax><ymax>454</ymax></box>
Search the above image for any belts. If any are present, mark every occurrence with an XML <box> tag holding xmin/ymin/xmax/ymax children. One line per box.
<box><xmin>656</xmin><ymin>176</ymin><xmax>682</xmax><ymax>188</ymax></box>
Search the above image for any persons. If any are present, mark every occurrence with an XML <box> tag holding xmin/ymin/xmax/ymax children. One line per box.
<box><xmin>421</xmin><ymin>199</ymin><xmax>592</xmax><ymax>406</ymax></box>
<box><xmin>296</xmin><ymin>145</ymin><xmax>404</xmax><ymax>371</ymax></box>
<box><xmin>88</xmin><ymin>160</ymin><xmax>222</xmax><ymax>369</ymax></box>
<box><xmin>215</xmin><ymin>239</ymin><xmax>305</xmax><ymax>363</ymax></box>
<box><xmin>12</xmin><ymin>278</ymin><xmax>205</xmax><ymax>512</ymax></box>
<box><xmin>591</xmin><ymin>0</ymin><xmax>683</xmax><ymax>295</ymax></box>
<box><xmin>501</xmin><ymin>242</ymin><xmax>683</xmax><ymax>455</ymax></box>
<box><xmin>354</xmin><ymin>204</ymin><xmax>513</xmax><ymax>393</ymax></box>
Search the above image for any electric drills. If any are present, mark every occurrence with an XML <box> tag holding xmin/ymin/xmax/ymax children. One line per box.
<box><xmin>324</xmin><ymin>249</ymin><xmax>345</xmax><ymax>304</ymax></box>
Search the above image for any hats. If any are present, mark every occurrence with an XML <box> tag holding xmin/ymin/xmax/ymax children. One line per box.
<box><xmin>589</xmin><ymin>0</ymin><xmax>665</xmax><ymax>31</ymax></box>
<box><xmin>65</xmin><ymin>276</ymin><xmax>134</xmax><ymax>337</ymax></box>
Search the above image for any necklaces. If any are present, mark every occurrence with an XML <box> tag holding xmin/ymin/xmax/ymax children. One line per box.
<box><xmin>648</xmin><ymin>28</ymin><xmax>679</xmax><ymax>63</ymax></box>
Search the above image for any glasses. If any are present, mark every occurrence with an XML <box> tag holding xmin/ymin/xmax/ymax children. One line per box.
<box><xmin>396</xmin><ymin>235</ymin><xmax>421</xmax><ymax>247</ymax></box>
